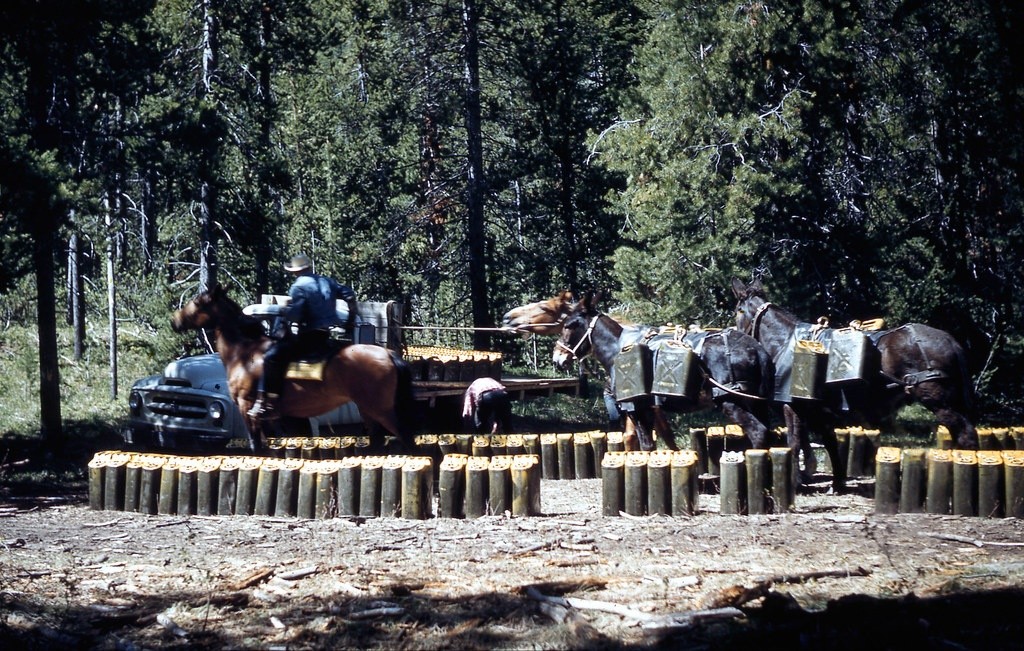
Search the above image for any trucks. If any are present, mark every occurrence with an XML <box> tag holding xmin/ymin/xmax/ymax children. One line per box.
<box><xmin>110</xmin><ymin>293</ymin><xmax>595</xmax><ymax>461</ymax></box>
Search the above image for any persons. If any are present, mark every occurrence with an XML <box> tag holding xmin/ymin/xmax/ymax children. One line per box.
<box><xmin>462</xmin><ymin>377</ymin><xmax>510</xmax><ymax>435</ymax></box>
<box><xmin>246</xmin><ymin>254</ymin><xmax>359</xmax><ymax>420</ymax></box>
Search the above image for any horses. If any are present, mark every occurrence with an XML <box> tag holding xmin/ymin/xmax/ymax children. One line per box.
<box><xmin>170</xmin><ymin>288</ymin><xmax>418</xmax><ymax>456</ymax></box>
<box><xmin>552</xmin><ymin>289</ymin><xmax>773</xmax><ymax>449</ymax></box>
<box><xmin>732</xmin><ymin>276</ymin><xmax>979</xmax><ymax>494</ymax></box>
<box><xmin>502</xmin><ymin>291</ymin><xmax>638</xmax><ymax>451</ymax></box>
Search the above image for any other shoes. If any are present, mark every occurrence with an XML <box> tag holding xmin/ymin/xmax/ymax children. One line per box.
<box><xmin>246</xmin><ymin>402</ymin><xmax>281</xmax><ymax>422</ymax></box>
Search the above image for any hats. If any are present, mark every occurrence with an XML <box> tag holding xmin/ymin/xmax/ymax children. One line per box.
<box><xmin>283</xmin><ymin>255</ymin><xmax>312</xmax><ymax>272</ymax></box>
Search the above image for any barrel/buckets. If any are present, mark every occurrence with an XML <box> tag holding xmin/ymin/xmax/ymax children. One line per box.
<box><xmin>650</xmin><ymin>339</ymin><xmax>693</xmax><ymax>397</ymax></box>
<box><xmin>825</xmin><ymin>327</ymin><xmax>871</xmax><ymax>384</ymax></box>
<box><xmin>789</xmin><ymin>346</ymin><xmax>828</xmax><ymax>400</ymax></box>
<box><xmin>615</xmin><ymin>344</ymin><xmax>651</xmax><ymax>402</ymax></box>
